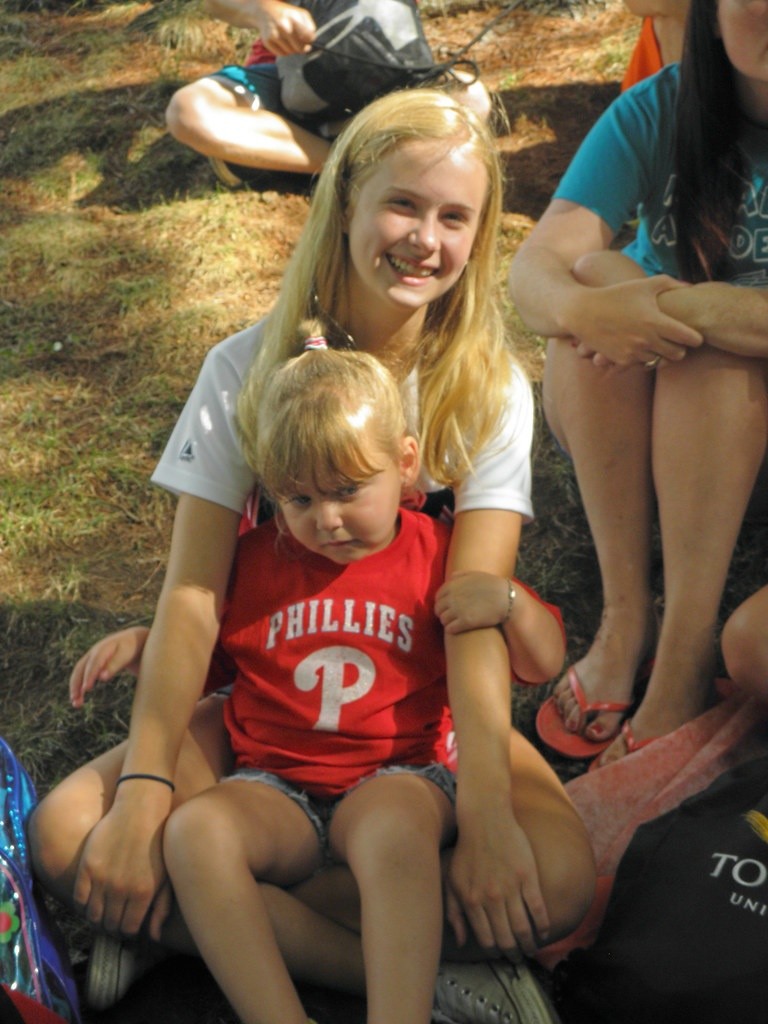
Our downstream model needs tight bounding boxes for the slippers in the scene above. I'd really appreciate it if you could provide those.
[588,717,666,778]
[535,663,635,760]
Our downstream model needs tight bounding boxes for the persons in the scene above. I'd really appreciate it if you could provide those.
[508,0,768,779]
[718,570,768,705]
[26,88,598,1024]
[65,326,570,1024]
[163,1,494,188]
[620,0,715,96]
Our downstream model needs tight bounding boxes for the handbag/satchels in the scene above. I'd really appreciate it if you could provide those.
[275,1,437,123]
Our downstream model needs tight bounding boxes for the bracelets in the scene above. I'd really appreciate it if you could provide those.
[109,768,180,791]
[501,574,515,628]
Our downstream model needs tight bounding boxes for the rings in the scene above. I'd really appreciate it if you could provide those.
[641,354,662,369]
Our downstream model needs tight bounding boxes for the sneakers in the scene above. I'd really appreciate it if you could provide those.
[82,932,141,1010]
[431,958,561,1022]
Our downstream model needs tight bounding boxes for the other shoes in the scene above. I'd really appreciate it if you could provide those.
[208,158,268,189]
